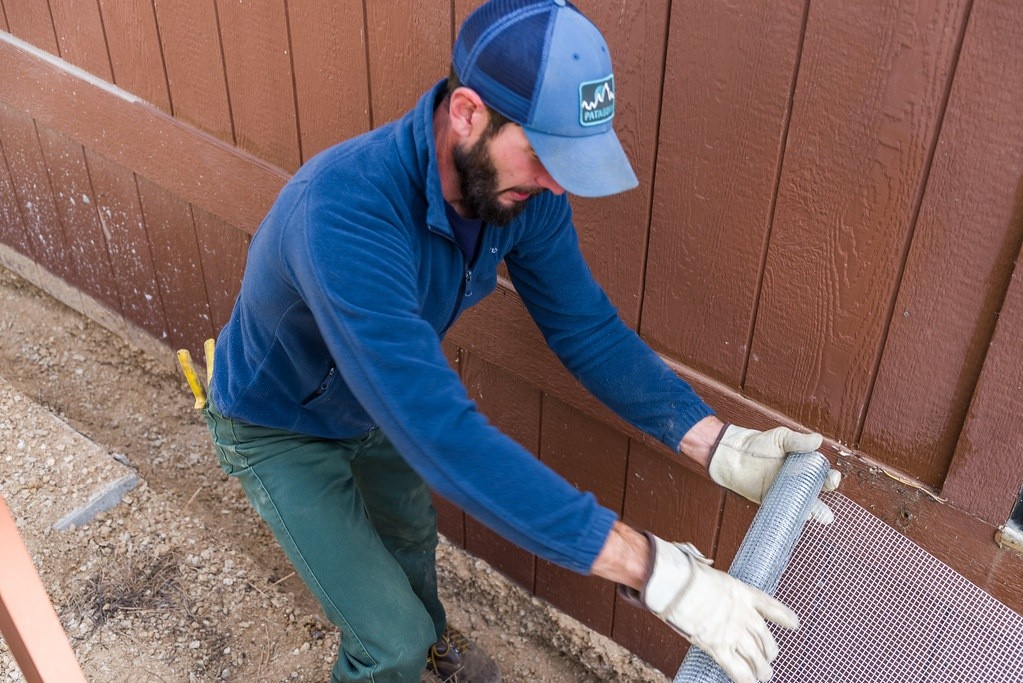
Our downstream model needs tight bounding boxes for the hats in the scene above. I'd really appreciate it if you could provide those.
[451,0,638,198]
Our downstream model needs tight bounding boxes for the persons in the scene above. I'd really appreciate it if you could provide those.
[203,0,841,683]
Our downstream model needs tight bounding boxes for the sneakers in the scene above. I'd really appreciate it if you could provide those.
[426,621,502,683]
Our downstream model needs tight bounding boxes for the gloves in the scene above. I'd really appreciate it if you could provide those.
[706,422,842,526]
[616,529,800,683]
